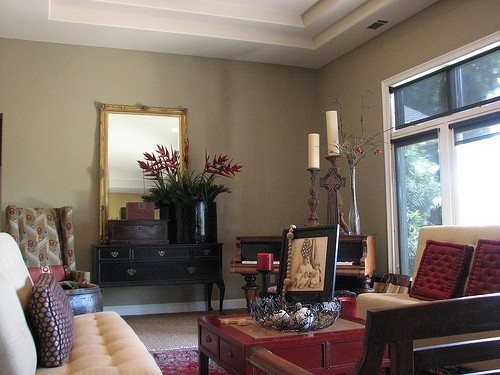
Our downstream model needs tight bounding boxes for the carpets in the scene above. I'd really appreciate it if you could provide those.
[148,347,228,375]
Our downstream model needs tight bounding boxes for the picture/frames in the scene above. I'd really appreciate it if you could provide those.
[280,224,340,301]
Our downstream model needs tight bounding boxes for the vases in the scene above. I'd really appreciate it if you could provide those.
[348,165,360,234]
[57,281,104,316]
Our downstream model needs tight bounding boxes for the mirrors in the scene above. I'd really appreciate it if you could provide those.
[97,103,188,244]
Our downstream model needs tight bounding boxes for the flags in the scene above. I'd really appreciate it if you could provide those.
[301,238,321,270]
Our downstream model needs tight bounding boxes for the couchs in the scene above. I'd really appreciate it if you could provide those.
[356,224,500,371]
[0,232,162,375]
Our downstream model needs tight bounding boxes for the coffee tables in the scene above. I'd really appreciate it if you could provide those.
[198,313,389,375]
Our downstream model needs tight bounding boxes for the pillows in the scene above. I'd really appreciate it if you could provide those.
[409,239,500,301]
[26,272,75,368]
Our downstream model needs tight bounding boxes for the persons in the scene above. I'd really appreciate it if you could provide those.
[294,258,323,289]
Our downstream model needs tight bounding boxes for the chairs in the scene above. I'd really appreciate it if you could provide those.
[6,205,90,285]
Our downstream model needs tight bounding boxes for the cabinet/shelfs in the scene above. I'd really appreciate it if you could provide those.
[90,243,226,316]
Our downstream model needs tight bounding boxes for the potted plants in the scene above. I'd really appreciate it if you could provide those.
[144,144,244,244]
[137,151,175,243]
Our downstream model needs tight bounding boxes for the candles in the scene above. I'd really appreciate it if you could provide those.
[326,111,339,156]
[308,133,320,168]
[256,253,274,270]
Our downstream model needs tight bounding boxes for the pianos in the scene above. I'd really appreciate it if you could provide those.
[230,233,377,313]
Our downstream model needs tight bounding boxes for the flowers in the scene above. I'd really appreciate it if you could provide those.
[334,94,394,233]
